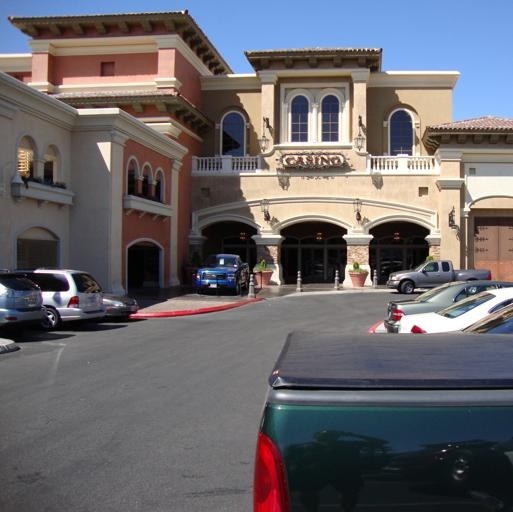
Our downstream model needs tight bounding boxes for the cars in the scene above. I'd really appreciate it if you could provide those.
[0,272,47,333]
[396,286,512,333]
[14,268,107,331]
[383,280,511,334]
[253,329,512,510]
[102,292,138,322]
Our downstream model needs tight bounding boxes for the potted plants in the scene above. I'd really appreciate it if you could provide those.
[348,262,369,288]
[252,256,273,285]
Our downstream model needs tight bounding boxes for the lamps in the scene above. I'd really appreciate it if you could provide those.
[260,199,271,221]
[353,199,362,220]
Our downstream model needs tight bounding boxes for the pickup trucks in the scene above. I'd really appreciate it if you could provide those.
[192,254,249,296]
[386,259,491,294]
